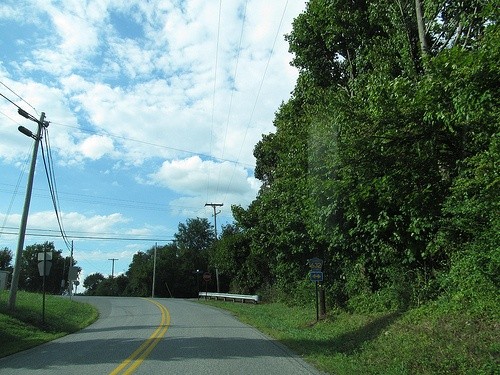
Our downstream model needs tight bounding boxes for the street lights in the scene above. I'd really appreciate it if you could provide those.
[6,109,49,310]
[150,242,159,297]
